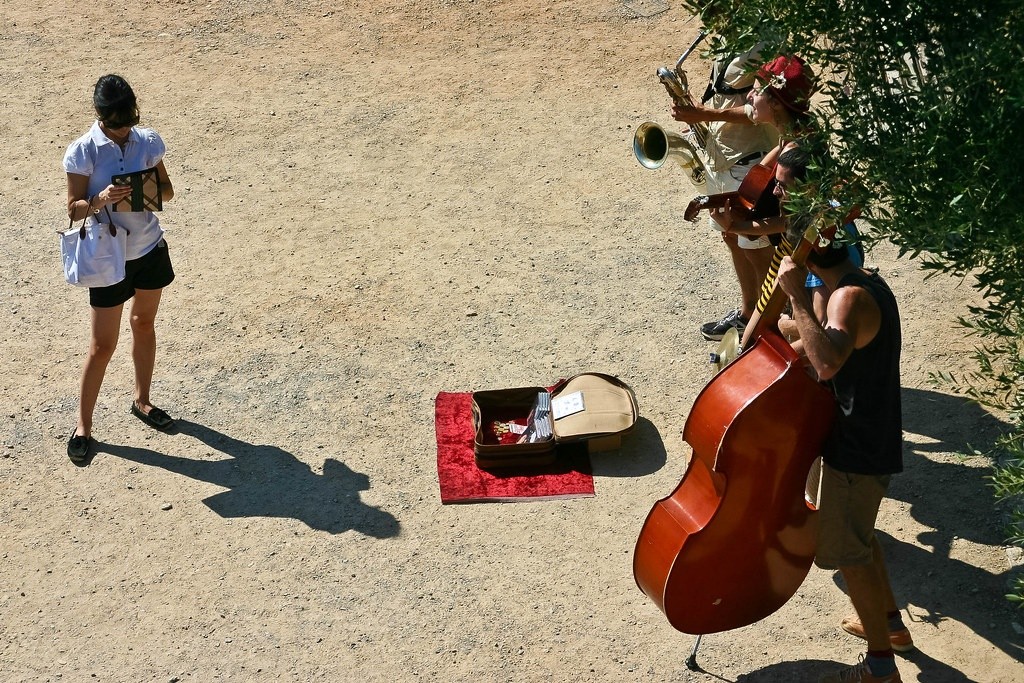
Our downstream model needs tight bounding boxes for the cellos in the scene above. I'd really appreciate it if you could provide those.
[630,175,872,668]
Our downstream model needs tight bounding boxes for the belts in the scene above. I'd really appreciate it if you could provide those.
[741,152,770,163]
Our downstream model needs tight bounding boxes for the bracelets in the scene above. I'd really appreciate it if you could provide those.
[725,221,735,234]
[88,196,100,214]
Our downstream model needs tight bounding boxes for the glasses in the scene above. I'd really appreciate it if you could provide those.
[773,179,791,193]
[101,109,142,129]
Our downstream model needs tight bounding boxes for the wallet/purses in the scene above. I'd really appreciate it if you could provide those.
[111,167,164,213]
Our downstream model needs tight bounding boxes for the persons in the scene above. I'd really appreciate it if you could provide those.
[671,19,788,358]
[709,54,865,507]
[777,202,913,683]
[63,73,176,463]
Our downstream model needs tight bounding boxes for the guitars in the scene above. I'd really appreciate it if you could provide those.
[683,162,780,247]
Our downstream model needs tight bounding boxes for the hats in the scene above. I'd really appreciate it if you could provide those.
[753,56,815,112]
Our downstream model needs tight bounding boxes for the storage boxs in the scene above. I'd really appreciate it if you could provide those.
[471,372,639,465]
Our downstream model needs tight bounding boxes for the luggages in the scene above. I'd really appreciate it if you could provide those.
[470,372,640,470]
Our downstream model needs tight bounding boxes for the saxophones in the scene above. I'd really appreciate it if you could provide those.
[632,28,710,195]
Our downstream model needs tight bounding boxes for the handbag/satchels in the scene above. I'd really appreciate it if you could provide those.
[57,193,131,282]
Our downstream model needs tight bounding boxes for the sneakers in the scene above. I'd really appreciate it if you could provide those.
[66,426,91,462]
[818,656,902,683]
[131,400,174,430]
[842,614,915,650]
[699,307,749,342]
